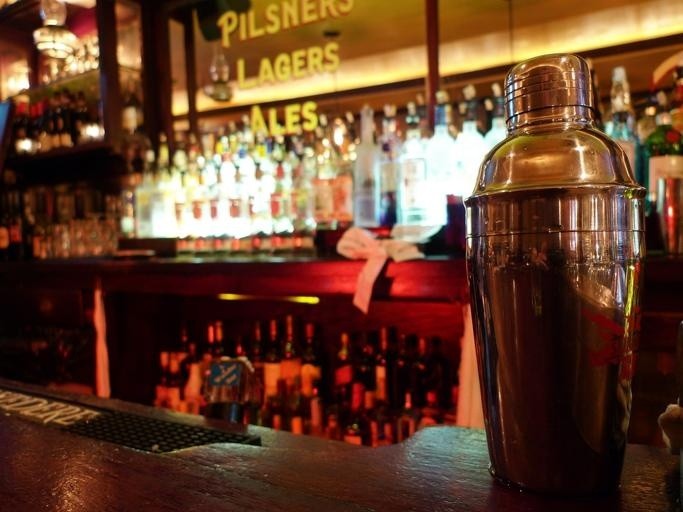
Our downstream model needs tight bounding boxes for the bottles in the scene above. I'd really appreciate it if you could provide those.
[131,306,462,451]
[459,53,647,499]
[115,58,682,240]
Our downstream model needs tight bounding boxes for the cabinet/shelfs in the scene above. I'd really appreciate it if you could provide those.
[0,0,683,447]
